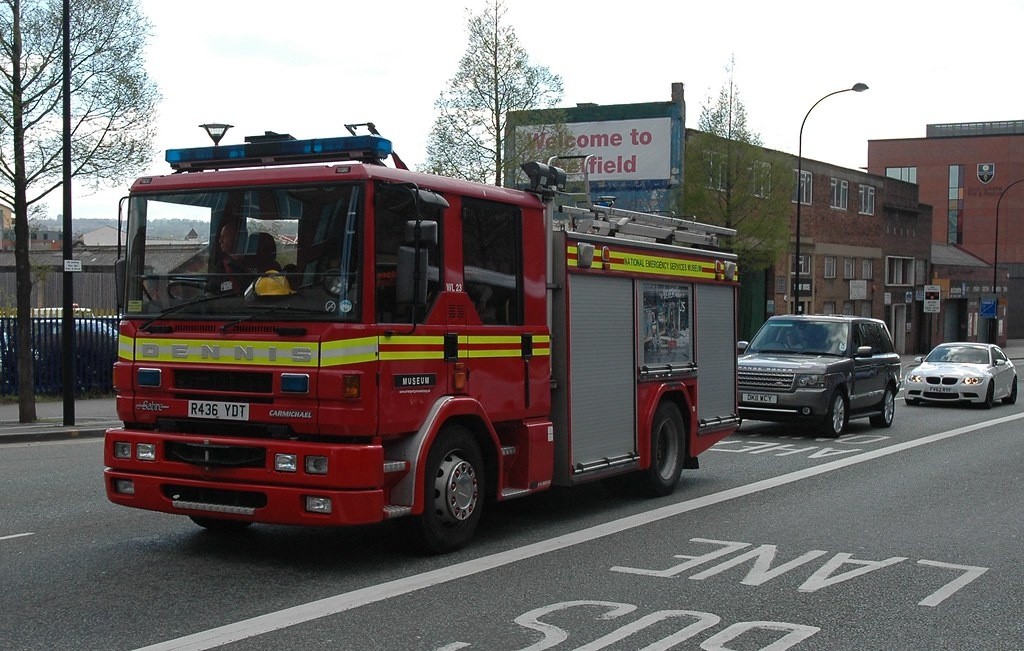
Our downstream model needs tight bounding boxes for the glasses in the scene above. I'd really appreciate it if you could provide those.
[787,334,794,336]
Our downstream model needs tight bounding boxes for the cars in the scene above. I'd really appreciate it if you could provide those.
[0,315,122,393]
[904,341,1017,409]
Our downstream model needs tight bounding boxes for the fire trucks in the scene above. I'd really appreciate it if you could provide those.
[102,132,742,550]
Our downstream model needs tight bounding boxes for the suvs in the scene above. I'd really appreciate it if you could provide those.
[738,314,902,439]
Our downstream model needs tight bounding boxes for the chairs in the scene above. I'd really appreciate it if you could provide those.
[247,232,282,272]
[464,230,486,268]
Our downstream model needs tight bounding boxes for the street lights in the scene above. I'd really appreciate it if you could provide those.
[199,123,234,172]
[795,82,868,315]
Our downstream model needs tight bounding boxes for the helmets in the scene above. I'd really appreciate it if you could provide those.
[255,271,297,296]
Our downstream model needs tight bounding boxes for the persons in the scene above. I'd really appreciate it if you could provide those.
[204,223,279,297]
[786,330,803,350]
